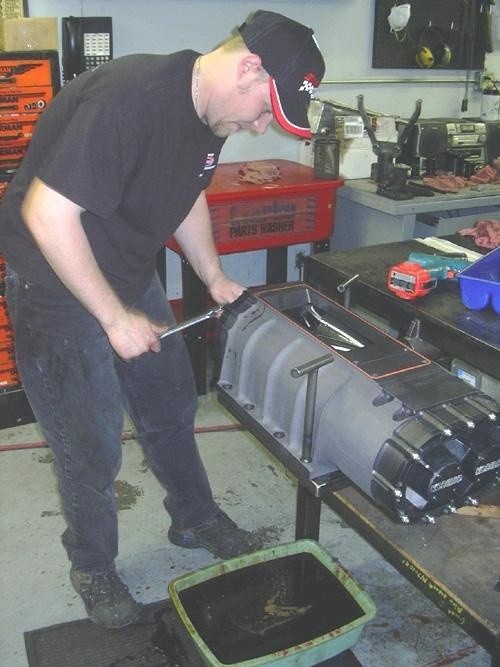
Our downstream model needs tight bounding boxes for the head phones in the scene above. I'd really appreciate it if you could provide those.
[416,25,452,68]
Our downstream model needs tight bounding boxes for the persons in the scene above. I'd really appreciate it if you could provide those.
[0,9,324,630]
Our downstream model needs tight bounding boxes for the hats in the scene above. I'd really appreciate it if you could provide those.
[238,9,325,140]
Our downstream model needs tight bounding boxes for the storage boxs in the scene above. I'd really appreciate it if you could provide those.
[167,538,374,666]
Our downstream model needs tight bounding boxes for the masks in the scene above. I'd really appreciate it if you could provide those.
[388,0,411,42]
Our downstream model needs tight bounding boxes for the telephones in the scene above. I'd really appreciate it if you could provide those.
[61,16,113,85]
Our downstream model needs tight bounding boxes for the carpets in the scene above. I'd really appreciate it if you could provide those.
[23,596,365,667]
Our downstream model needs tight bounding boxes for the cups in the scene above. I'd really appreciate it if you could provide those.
[315,140,340,180]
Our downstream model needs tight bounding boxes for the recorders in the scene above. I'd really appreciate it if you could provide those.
[396,116,500,179]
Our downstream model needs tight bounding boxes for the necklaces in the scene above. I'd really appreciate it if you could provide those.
[192,53,198,117]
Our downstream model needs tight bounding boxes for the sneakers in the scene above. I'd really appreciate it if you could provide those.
[70,561,142,631]
[168,508,262,560]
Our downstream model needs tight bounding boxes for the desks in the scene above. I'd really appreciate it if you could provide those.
[156,157,345,396]
[337,180,500,250]
[306,233,500,379]
